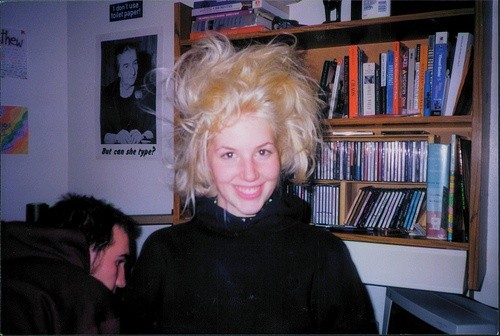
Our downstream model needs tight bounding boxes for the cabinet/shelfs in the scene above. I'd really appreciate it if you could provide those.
[173,0,484,288]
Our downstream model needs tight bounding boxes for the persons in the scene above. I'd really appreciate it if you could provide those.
[124,31,379,336]
[0,193,138,336]
[100,44,155,145]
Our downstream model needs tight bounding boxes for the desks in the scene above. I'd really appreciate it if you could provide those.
[380,286,500,336]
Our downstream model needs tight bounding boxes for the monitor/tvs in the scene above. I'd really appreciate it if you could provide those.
[382,286,500,335]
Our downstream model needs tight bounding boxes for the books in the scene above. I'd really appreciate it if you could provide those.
[287,135,470,241]
[317,32,473,117]
[191,1,290,35]
